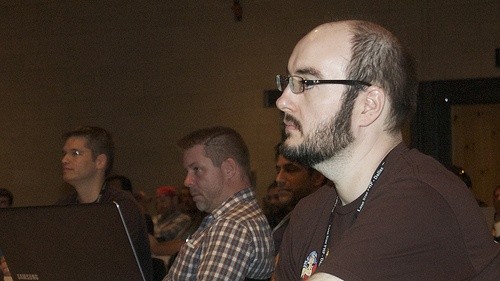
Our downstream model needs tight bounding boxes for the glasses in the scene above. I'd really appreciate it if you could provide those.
[275,73,373,96]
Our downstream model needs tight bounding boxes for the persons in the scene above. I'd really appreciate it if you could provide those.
[272,20,500,281]
[0,126,500,281]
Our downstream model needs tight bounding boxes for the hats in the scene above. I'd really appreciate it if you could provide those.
[155,187,180,198]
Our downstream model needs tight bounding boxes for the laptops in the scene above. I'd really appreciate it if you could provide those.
[0,201,146,281]
